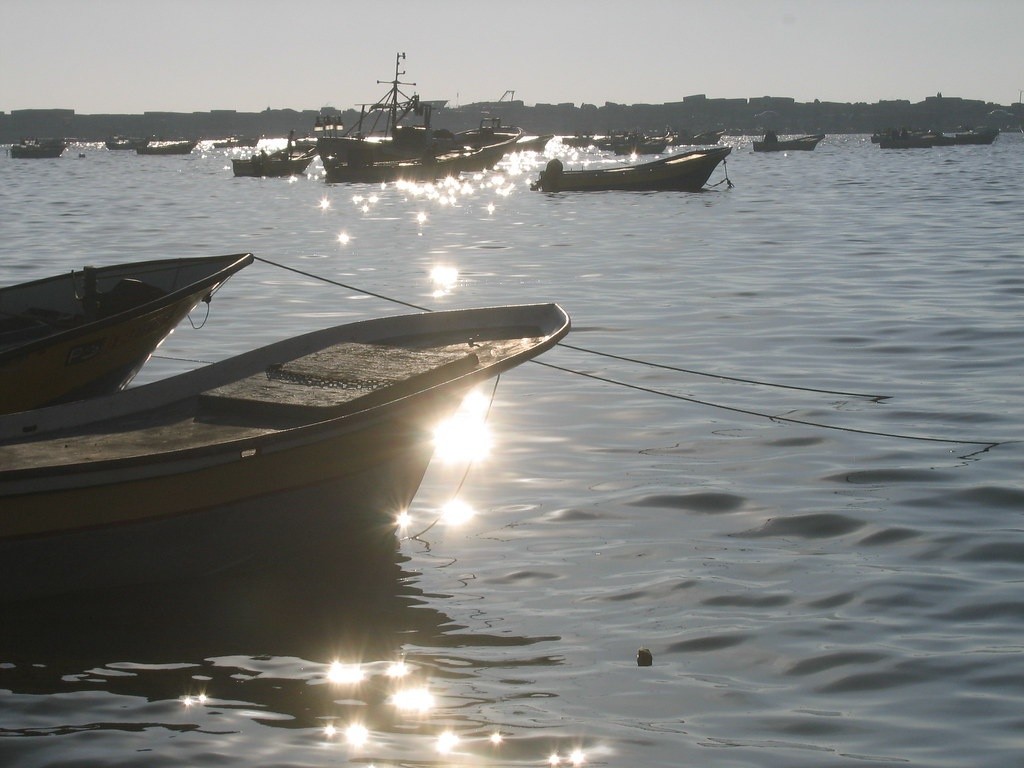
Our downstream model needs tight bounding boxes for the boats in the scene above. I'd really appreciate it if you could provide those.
[0,249,257,414]
[135,134,203,155]
[507,132,555,153]
[752,130,826,152]
[211,132,261,149]
[560,123,728,157]
[104,134,150,150]
[311,53,525,183]
[868,121,1001,149]
[527,146,733,193]
[9,136,71,158]
[230,128,319,178]
[0,297,574,545]
